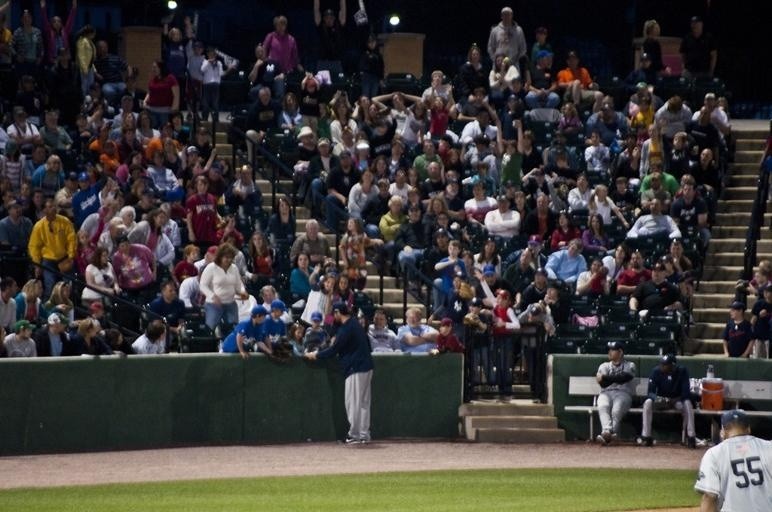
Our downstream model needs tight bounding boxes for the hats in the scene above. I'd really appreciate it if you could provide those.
[48,313,69,325]
[15,320,36,331]
[727,301,745,308]
[482,264,494,276]
[662,353,676,365]
[79,320,92,331]
[252,306,267,314]
[468,297,483,306]
[495,289,509,298]
[312,312,322,322]
[607,341,620,349]
[722,410,749,426]
[527,235,540,245]
[271,300,288,310]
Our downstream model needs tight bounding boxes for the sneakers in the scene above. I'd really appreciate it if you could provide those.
[339,436,368,445]
[636,436,654,447]
[597,432,619,446]
[683,437,707,448]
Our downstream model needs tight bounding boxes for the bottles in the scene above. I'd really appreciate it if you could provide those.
[706,364,715,377]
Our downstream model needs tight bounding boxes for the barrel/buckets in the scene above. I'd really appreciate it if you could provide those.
[700,377,726,411]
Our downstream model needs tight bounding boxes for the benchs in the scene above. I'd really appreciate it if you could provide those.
[564,376,771,445]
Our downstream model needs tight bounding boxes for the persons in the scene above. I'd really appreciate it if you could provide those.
[694,409,772,512]
[1,0,772,448]
